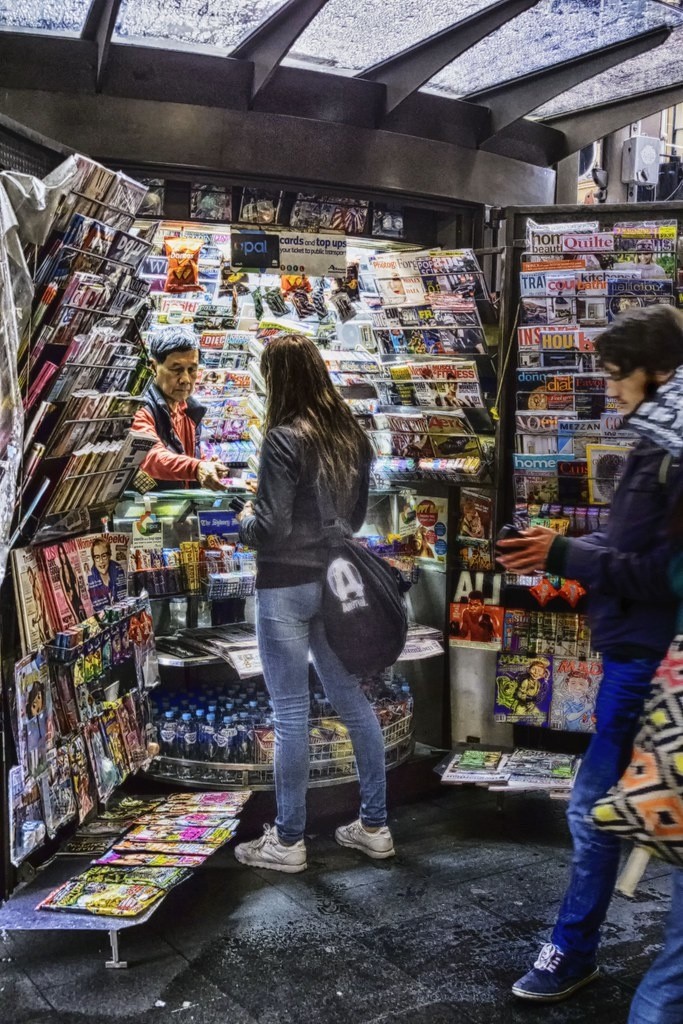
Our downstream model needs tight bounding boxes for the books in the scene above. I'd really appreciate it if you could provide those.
[1,151,255,920]
[255,215,683,801]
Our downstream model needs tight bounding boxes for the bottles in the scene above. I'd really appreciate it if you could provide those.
[151,670,413,763]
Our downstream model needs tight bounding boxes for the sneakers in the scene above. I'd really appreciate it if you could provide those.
[333,817,397,860]
[232,823,309,874]
[511,942,602,1003]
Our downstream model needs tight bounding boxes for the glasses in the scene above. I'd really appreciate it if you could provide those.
[607,370,632,381]
[91,552,108,560]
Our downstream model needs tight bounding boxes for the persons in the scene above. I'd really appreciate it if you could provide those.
[626,865,683,1024]
[130,326,232,493]
[233,334,396,874]
[495,302,682,1004]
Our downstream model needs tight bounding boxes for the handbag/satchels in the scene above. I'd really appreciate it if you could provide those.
[319,531,411,676]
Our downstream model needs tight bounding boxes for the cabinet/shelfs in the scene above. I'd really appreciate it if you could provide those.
[111,476,462,823]
